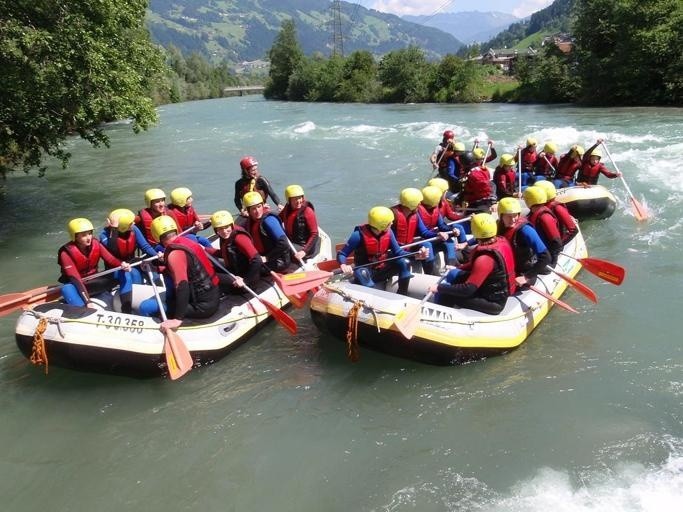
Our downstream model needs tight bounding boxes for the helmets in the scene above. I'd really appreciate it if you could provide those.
[286,185,304,200]
[500,154,515,166]
[544,144,554,153]
[368,207,394,231]
[151,216,177,242]
[444,131,453,138]
[524,186,547,208]
[498,197,521,215]
[212,211,234,230]
[471,214,497,239]
[534,181,556,200]
[528,138,536,145]
[145,189,166,208]
[171,188,192,207]
[401,188,423,210]
[422,187,442,207]
[240,157,257,169]
[572,146,584,156]
[453,144,464,151]
[473,149,484,159]
[591,150,601,157]
[69,218,95,242]
[429,179,448,192]
[243,192,263,207]
[109,209,134,233]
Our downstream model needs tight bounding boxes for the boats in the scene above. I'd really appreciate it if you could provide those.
[14,224,333,380]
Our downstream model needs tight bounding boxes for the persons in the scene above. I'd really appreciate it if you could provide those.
[197,210,264,294]
[233,155,285,226]
[234,191,290,276]
[99,208,164,287]
[335,132,621,315]
[133,188,183,253]
[167,187,214,248]
[139,216,221,334]
[264,184,319,262]
[56,217,133,313]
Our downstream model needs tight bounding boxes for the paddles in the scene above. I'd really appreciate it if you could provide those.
[198,206,281,225]
[262,261,307,308]
[145,263,193,380]
[0,266,124,317]
[286,234,328,295]
[558,252,625,286]
[599,140,648,221]
[393,268,450,340]
[546,265,596,303]
[281,248,428,296]
[317,234,442,272]
[204,250,296,335]
[523,283,579,315]
[20,253,164,312]
